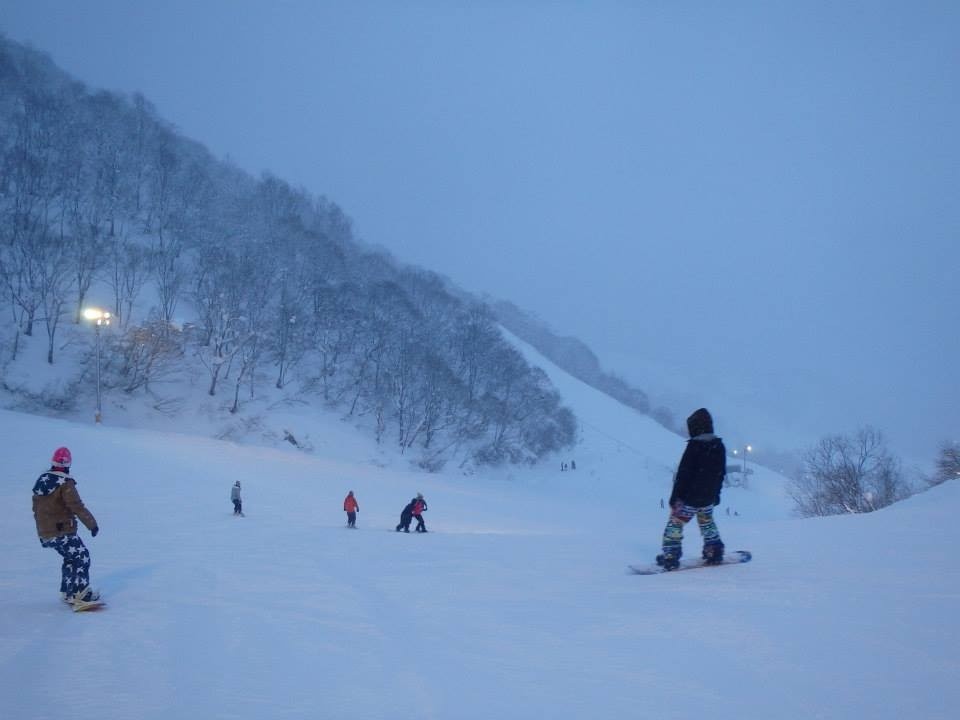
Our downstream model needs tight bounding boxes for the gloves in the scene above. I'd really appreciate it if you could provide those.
[713,496,720,507]
[92,527,99,537]
[669,496,677,506]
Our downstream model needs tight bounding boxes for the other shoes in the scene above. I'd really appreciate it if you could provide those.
[89,592,101,601]
[655,555,679,570]
[702,550,723,564]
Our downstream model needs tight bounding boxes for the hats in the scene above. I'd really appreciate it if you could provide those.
[49,447,72,469]
[235,480,241,487]
[416,491,424,498]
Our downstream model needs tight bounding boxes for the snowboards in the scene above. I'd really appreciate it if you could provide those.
[627,550,751,575]
[63,597,107,612]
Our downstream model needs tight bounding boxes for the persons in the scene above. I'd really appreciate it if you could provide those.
[396,494,428,532]
[33,447,100,603]
[344,491,359,528]
[656,408,726,566]
[231,480,242,513]
